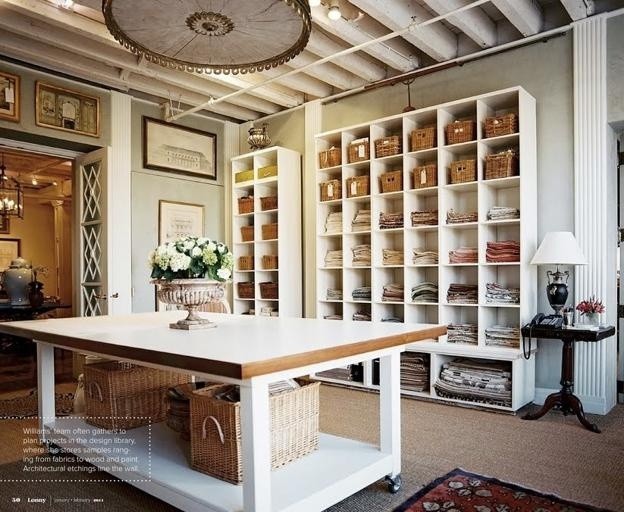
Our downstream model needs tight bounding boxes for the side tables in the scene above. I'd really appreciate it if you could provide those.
[521,326,615,434]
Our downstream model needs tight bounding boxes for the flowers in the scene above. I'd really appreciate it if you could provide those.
[31,262,48,280]
[149,234,234,282]
[576,295,606,315]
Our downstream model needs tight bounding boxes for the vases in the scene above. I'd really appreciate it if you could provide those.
[28,280,44,308]
[150,277,227,332]
[582,314,603,327]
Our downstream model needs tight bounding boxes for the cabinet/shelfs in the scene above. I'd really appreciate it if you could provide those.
[230,147,302,318]
[313,86,539,420]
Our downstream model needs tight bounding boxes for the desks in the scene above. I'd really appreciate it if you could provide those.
[0,312,446,509]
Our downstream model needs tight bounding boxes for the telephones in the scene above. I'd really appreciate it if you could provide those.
[524,313,564,360]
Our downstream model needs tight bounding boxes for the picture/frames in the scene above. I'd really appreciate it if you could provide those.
[0,238,22,258]
[141,113,218,180]
[157,199,206,243]
[1,216,10,234]
[34,80,101,138]
[0,70,22,123]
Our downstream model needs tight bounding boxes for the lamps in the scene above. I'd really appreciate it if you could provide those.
[328,0,341,19]
[100,0,311,75]
[531,230,585,315]
[0,170,25,219]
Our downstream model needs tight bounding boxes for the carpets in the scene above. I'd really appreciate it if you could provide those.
[390,466,606,512]
